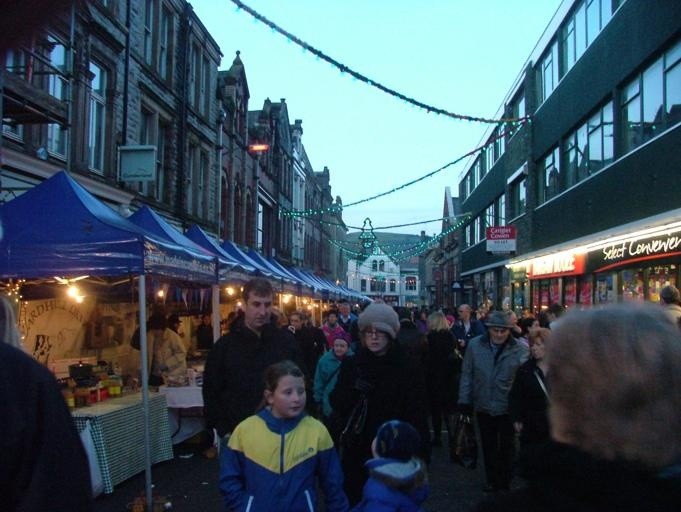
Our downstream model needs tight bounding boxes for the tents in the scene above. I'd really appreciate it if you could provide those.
[1,170,221,512]
[116,204,376,324]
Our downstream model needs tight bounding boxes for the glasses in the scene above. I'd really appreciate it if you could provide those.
[364,330,387,337]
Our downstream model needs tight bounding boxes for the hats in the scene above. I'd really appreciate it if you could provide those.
[660,286,680,299]
[484,310,515,329]
[333,330,352,347]
[358,299,400,339]
[375,421,422,462]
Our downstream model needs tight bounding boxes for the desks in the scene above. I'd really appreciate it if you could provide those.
[66,391,175,497]
[160,385,207,442]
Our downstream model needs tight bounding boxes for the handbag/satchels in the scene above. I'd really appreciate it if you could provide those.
[452,414,478,469]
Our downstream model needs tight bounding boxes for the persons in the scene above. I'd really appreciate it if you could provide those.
[147,317,187,386]
[131,311,163,351]
[656,287,679,322]
[338,302,359,333]
[474,303,679,511]
[351,421,428,511]
[330,299,428,507]
[443,302,480,348]
[168,315,185,339]
[509,327,553,506]
[202,279,301,451]
[1,300,93,507]
[422,310,459,450]
[196,314,213,345]
[217,363,349,512]
[279,311,314,345]
[486,294,576,338]
[321,310,351,350]
[312,332,357,452]
[391,302,424,354]
[459,310,530,501]
